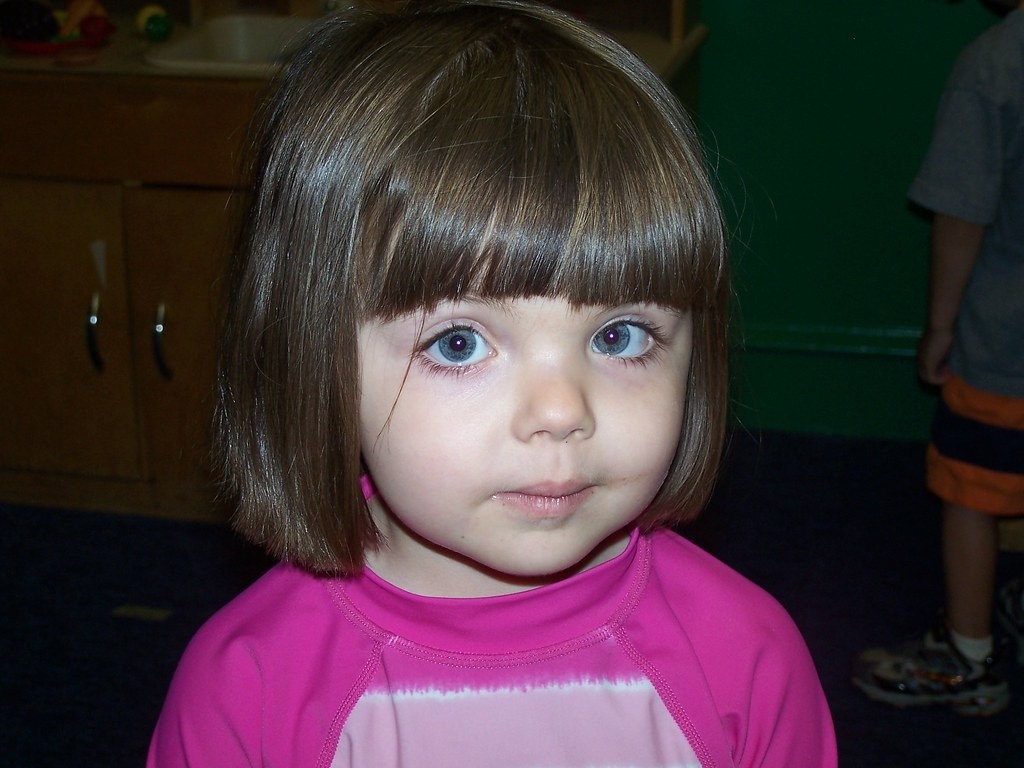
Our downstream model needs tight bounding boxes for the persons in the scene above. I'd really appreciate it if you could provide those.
[140,0,839,768]
[852,0,1024,719]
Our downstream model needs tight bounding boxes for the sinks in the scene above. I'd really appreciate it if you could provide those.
[126,12,331,79]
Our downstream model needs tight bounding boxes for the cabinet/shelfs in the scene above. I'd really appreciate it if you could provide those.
[1,68,270,522]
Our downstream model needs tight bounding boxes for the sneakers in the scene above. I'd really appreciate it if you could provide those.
[995,578,1024,652]
[843,621,1012,721]
